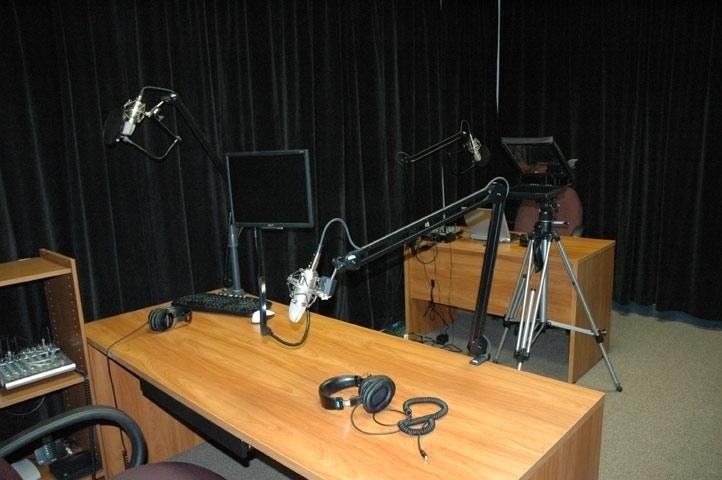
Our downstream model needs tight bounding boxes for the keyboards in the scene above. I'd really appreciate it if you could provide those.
[172,291,272,316]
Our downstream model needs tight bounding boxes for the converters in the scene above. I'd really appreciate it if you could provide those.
[436,334,448,344]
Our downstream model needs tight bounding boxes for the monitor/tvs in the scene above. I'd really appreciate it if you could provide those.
[224,149,317,231]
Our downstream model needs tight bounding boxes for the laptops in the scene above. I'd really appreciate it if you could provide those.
[461,206,520,243]
[500,136,575,201]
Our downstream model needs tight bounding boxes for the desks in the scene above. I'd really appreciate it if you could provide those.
[404,236,619,382]
[84,285,606,480]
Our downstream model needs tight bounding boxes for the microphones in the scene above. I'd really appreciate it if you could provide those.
[468,133,481,162]
[290,245,323,323]
[118,94,144,136]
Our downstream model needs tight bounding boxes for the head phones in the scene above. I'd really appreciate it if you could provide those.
[148,306,192,331]
[320,374,395,414]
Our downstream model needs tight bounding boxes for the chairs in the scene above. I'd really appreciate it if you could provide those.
[515,185,584,239]
[1,404,230,479]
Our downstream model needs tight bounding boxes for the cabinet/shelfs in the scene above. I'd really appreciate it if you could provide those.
[1,241,111,479]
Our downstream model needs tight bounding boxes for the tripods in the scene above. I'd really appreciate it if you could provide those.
[494,199,622,392]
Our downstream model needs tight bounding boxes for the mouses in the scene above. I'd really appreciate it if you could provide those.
[250,309,275,323]
